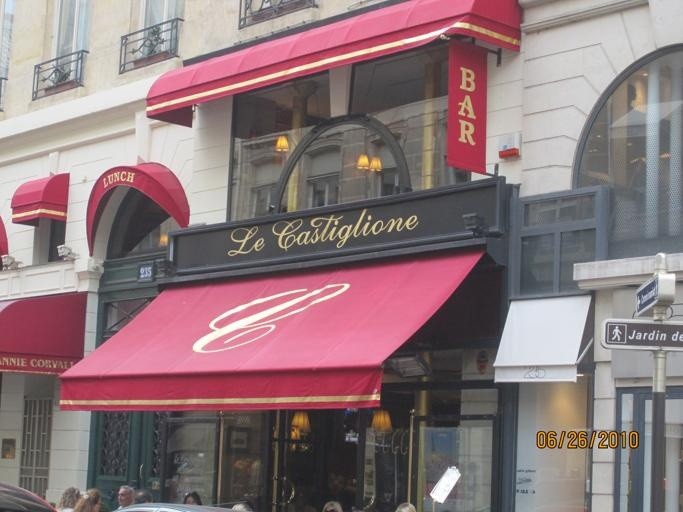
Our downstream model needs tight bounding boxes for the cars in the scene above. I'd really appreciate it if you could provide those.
[113,503,249,512]
[0,479,57,512]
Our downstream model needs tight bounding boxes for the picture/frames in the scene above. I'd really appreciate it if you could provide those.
[226,424,254,453]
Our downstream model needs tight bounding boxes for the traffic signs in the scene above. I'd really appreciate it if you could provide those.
[634,269,674,314]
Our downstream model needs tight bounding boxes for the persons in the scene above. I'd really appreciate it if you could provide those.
[57,486,80,512]
[74,488,101,512]
[183,491,201,505]
[116,484,134,509]
[395,502,416,512]
[132,488,152,503]
[232,502,253,512]
[321,500,343,511]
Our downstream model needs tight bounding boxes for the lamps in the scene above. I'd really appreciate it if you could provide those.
[356,129,384,173]
[1,255,19,272]
[276,135,289,163]
[56,245,78,260]
